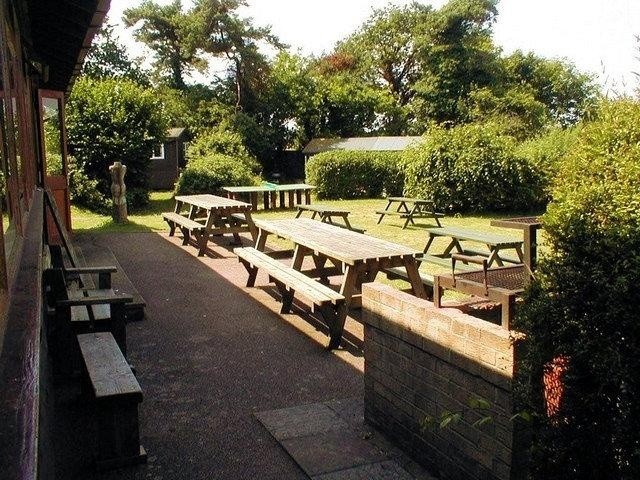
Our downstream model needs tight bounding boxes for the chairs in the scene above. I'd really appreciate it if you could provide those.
[48,243,134,354]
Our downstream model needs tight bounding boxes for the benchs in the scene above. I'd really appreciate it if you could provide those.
[77,331,148,464]
